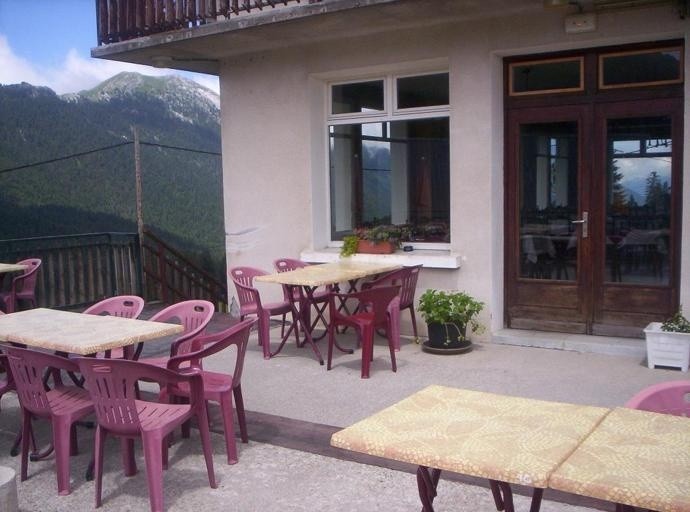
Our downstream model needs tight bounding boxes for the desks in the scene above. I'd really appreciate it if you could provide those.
[330,383,611,512]
[547,406,690,512]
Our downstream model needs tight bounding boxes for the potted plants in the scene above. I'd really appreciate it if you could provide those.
[416,289,489,355]
[642,303,690,372]
[339,224,403,257]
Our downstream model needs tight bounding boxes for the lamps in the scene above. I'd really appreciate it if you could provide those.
[543,0,598,34]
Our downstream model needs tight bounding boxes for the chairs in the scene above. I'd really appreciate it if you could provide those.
[624,381,690,419]
